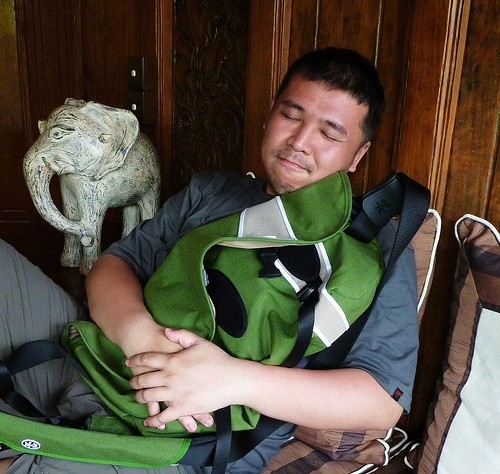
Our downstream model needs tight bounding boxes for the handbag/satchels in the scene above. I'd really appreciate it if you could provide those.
[0,170,432,474]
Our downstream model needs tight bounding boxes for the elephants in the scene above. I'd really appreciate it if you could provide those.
[23,97,161,275]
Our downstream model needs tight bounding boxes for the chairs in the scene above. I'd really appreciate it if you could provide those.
[403,211,500,474]
[256,206,444,474]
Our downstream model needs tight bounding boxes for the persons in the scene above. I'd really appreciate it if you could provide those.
[1,46,419,474]
